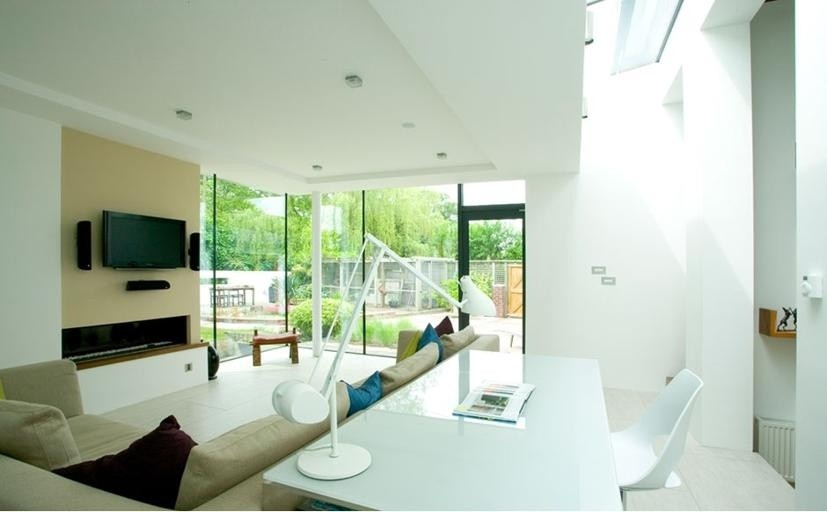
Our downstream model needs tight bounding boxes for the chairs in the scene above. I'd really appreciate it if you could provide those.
[608,367,704,511]
[215,286,243,307]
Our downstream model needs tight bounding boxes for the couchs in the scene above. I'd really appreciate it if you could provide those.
[0,328,500,510]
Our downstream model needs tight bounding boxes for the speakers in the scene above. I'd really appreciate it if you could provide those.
[127,281,171,291]
[188,232,201,271]
[77,220,92,270]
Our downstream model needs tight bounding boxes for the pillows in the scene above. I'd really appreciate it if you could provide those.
[397,316,456,366]
[338,369,383,418]
[51,413,200,510]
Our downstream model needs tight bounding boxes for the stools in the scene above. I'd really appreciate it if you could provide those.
[251,326,300,366]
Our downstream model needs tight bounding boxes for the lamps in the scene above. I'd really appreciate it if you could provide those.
[272,232,499,482]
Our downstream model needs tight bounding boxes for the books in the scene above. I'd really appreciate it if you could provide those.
[451,381,537,423]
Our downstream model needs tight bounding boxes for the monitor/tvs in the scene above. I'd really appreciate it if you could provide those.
[102,210,187,271]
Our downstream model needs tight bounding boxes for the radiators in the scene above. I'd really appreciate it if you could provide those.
[756,416,798,485]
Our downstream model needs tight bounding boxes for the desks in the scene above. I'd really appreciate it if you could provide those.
[259,349,625,510]
[210,288,254,308]
